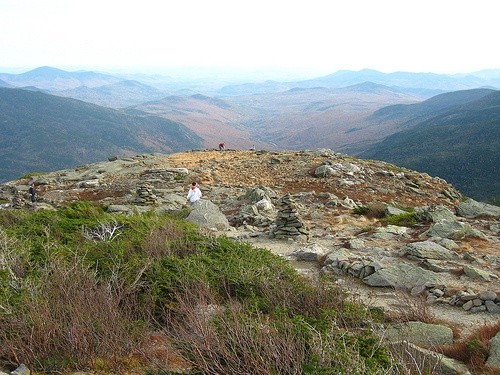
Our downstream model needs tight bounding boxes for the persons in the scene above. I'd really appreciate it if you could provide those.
[249,145,256,151]
[218,143,225,151]
[187,182,203,207]
[29,182,36,202]
